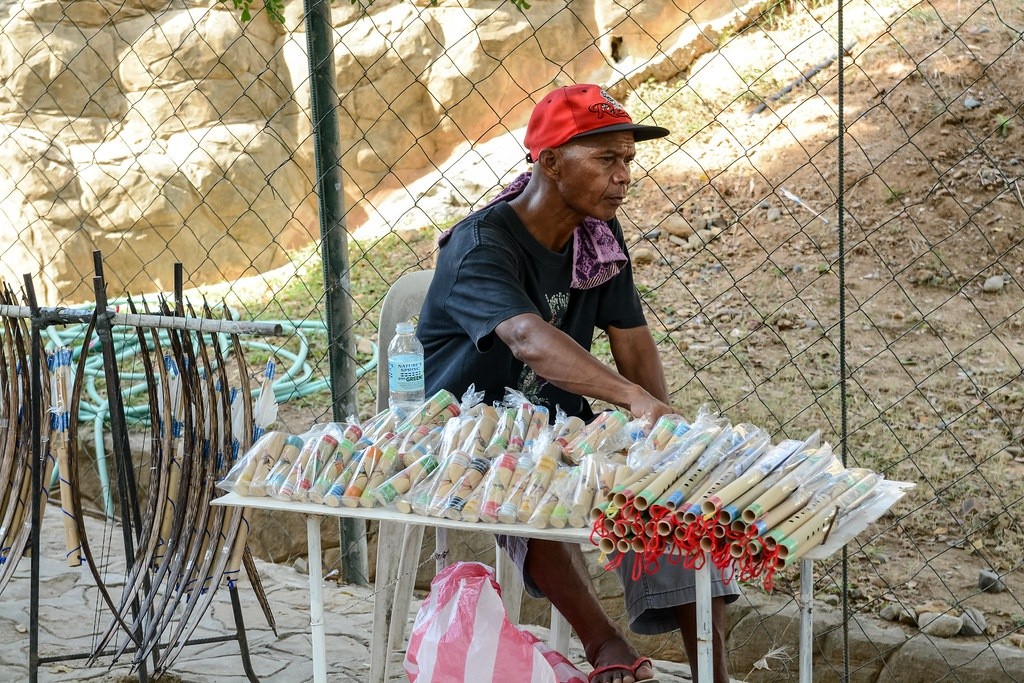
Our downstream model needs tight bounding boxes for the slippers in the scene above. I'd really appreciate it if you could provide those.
[589,657,659,683]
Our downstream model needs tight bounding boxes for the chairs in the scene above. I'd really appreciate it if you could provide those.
[368,269,571,683]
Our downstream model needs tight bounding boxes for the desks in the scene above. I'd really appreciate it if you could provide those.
[208,478,914,683]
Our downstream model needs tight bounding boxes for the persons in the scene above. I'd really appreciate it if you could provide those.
[416,83,741,683]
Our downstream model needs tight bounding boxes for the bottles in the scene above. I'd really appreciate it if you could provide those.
[387,322,426,420]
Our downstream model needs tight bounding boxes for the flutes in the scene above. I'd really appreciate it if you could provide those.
[586,420,883,571]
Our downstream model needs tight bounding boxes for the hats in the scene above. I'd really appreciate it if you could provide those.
[524,84,670,164]
